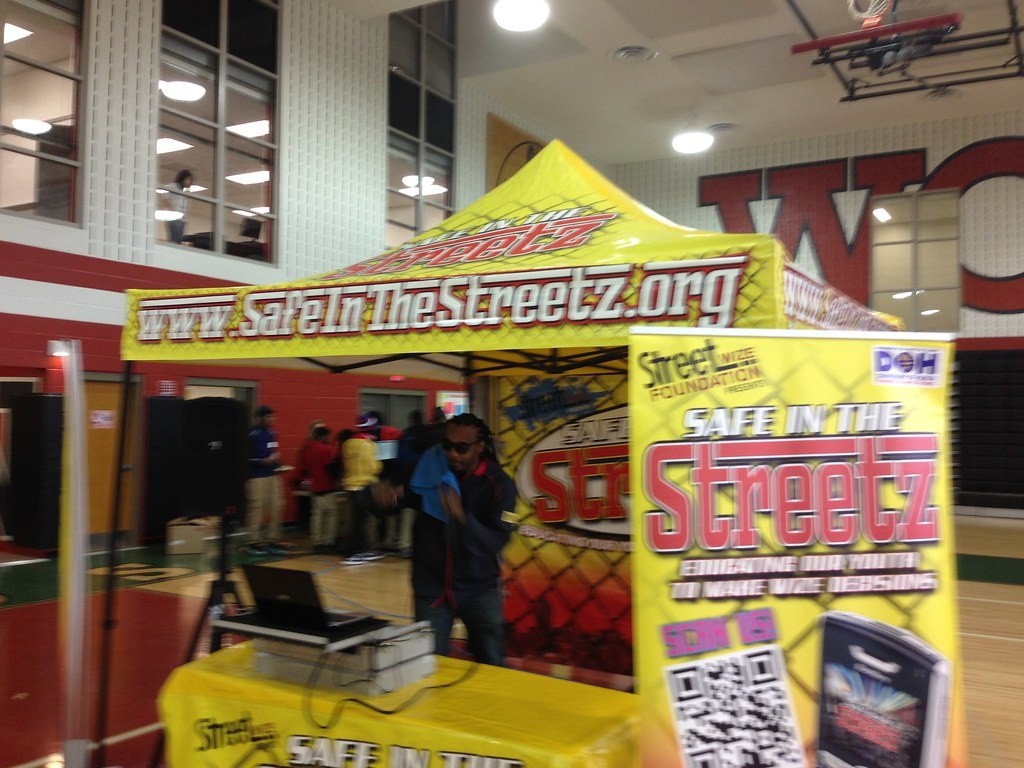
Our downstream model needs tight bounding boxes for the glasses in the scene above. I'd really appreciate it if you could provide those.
[441,437,480,455]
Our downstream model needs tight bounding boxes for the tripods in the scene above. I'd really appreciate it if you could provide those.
[147,516,249,767]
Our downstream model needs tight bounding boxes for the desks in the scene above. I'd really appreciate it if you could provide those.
[171,637,641,768]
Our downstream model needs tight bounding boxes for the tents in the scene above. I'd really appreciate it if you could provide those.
[78,137,908,768]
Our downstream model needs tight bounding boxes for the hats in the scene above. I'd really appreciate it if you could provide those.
[310,419,331,438]
[380,425,403,441]
[355,415,379,428]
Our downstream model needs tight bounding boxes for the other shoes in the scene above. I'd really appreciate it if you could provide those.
[310,541,410,565]
[268,539,300,554]
[237,542,269,557]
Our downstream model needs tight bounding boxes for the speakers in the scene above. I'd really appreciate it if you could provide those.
[171,395,250,513]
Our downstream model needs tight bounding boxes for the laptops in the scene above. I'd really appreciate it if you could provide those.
[242,564,373,631]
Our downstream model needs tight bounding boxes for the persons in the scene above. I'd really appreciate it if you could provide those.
[405,413,521,668]
[298,406,404,568]
[162,168,194,247]
[395,408,436,557]
[430,406,451,441]
[234,404,294,548]
